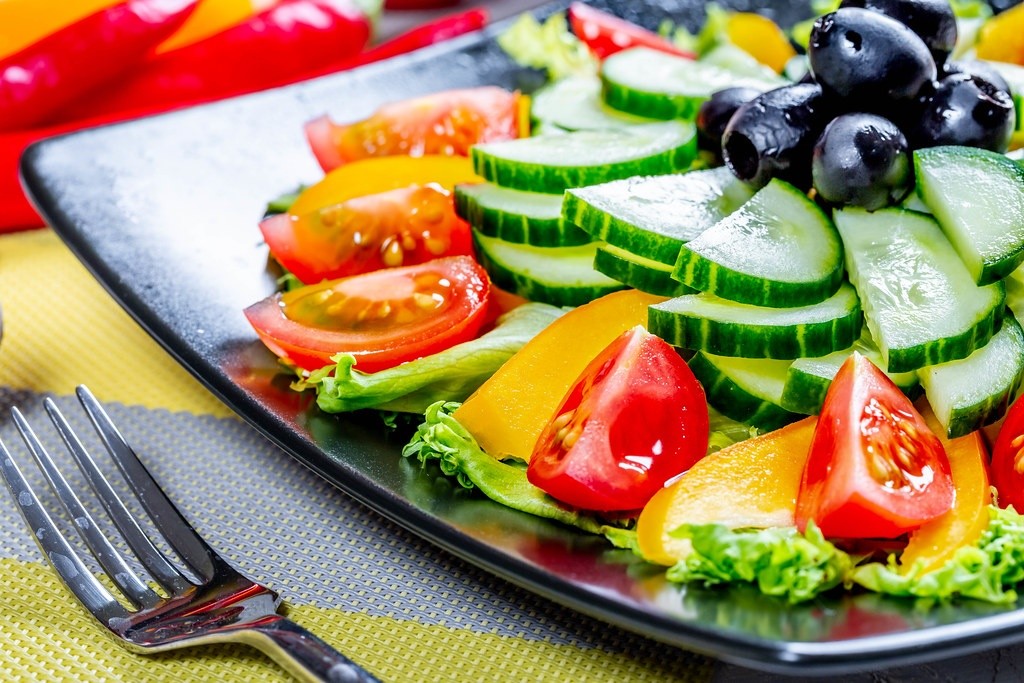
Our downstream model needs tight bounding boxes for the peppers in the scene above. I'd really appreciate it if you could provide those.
[0,0,487,235]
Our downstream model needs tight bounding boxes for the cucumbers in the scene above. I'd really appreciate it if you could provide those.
[454,35,1024,439]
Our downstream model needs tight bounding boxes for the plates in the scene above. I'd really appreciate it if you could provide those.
[17,1,1024,677]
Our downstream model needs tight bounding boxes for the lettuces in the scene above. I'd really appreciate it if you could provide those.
[665,486,1024,605]
[279,303,582,429]
[403,400,767,549]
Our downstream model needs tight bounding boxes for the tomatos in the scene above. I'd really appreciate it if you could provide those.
[794,350,955,538]
[258,182,476,285]
[990,393,1024,517]
[244,254,493,375]
[304,85,522,175]
[526,323,709,512]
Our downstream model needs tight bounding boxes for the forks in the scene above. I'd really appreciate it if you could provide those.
[1,384,388,682]
